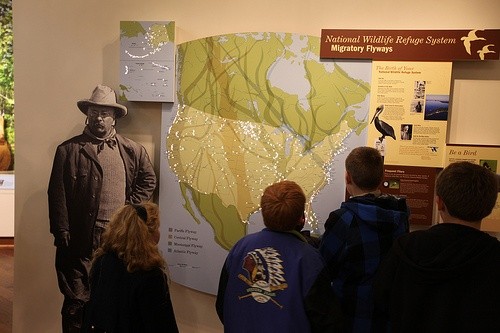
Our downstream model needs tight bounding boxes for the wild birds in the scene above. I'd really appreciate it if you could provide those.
[370,104,396,142]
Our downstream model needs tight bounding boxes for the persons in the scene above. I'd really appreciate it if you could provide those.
[312,146,413,333]
[410,160,500,333]
[214,181,338,333]
[46,85,159,333]
[88,202,181,333]
